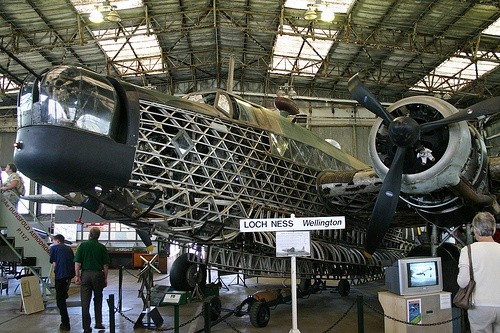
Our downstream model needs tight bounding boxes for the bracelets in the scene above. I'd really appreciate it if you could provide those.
[76,275,80,277]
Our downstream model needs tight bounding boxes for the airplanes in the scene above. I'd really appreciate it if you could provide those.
[7,62,500,327]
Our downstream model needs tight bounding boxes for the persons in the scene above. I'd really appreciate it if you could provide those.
[73,226,110,333]
[49,234,75,331]
[2,164,21,211]
[457,212,500,333]
[0,166,2,187]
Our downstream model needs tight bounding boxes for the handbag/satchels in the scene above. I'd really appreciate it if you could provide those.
[453,280,476,310]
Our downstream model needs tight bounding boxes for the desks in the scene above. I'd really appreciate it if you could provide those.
[107,251,148,270]
[141,255,158,267]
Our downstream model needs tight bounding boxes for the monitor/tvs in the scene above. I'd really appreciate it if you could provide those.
[384,256,444,296]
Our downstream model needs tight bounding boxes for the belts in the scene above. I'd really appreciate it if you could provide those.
[83,269,101,272]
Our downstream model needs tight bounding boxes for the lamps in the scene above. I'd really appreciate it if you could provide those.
[88,0,121,24]
[303,0,335,22]
[434,90,452,100]
[277,85,298,96]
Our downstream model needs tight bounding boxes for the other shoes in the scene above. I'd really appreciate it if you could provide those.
[84,328,92,333]
[95,323,106,329]
[60,323,70,331]
